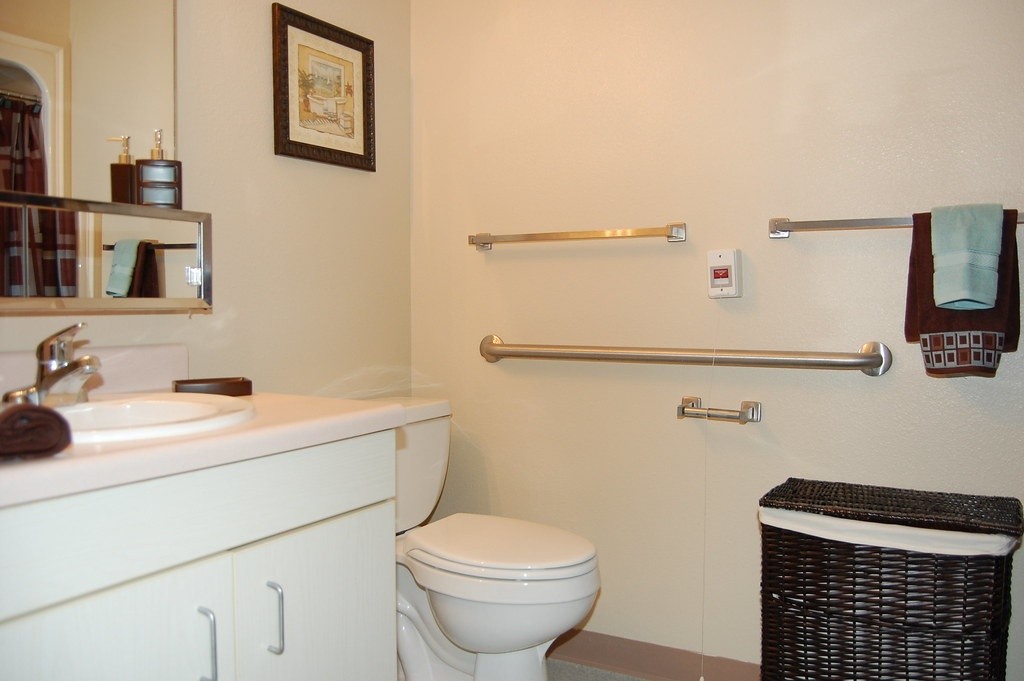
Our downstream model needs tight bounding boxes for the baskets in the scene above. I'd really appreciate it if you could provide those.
[758,478,1024,681]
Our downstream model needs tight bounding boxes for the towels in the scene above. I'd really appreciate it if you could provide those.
[127,240,161,298]
[930,202,1004,312]
[105,238,140,298]
[0,403,72,460]
[904,209,1020,378]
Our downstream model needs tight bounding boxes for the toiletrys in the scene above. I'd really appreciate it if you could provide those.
[107,134,136,202]
[136,127,181,211]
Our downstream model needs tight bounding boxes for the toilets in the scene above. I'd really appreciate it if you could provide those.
[368,395,602,679]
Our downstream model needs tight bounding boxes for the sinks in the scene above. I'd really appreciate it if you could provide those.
[54,399,218,430]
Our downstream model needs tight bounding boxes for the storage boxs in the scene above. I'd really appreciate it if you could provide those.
[756,477,1024,681]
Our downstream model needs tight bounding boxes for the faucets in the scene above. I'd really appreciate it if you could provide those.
[1,321,99,407]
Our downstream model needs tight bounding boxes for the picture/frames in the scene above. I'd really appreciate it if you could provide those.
[272,1,376,171]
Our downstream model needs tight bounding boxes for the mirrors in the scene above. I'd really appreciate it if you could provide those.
[1,189,215,314]
[1,0,178,206]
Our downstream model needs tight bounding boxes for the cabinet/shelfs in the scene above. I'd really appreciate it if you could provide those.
[0,495,397,680]
